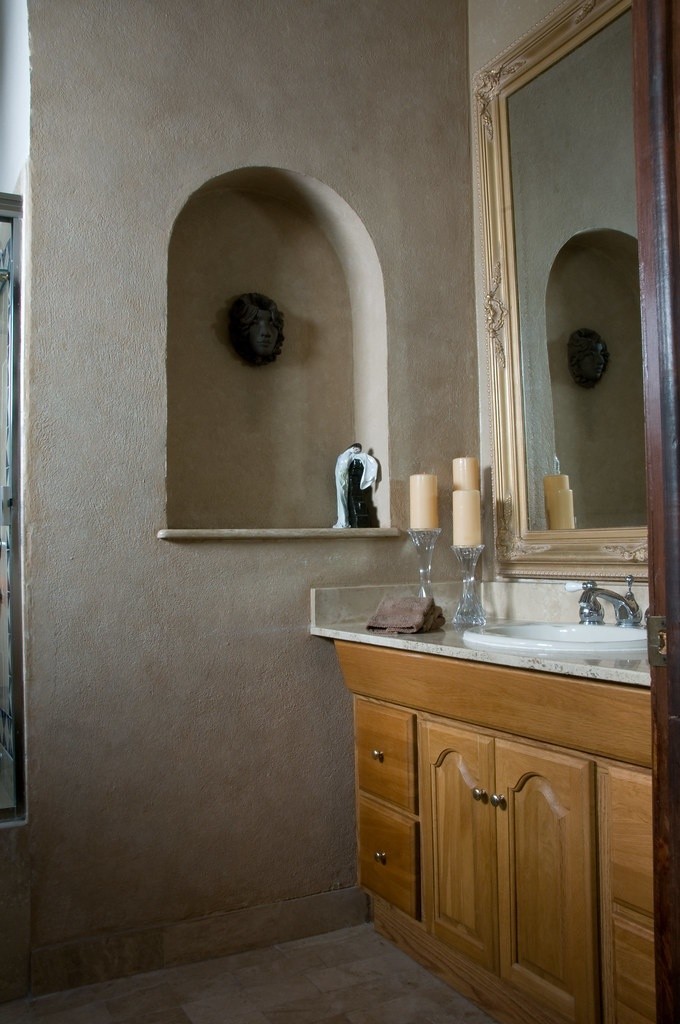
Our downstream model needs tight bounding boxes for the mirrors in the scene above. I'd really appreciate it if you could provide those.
[472,0,646,582]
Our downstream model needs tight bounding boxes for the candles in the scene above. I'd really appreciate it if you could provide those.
[453,454,480,491]
[409,471,439,531]
[452,490,482,548]
[543,453,576,529]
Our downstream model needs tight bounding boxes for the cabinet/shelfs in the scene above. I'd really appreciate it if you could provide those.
[308,618,658,1024]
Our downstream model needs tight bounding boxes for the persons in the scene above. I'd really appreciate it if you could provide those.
[568,326,610,389]
[228,292,286,367]
[333,443,379,529]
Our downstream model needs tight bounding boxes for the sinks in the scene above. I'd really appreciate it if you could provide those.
[463,622,648,652]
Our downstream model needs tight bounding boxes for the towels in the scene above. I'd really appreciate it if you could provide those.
[366,597,446,634]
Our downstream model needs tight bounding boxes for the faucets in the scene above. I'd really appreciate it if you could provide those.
[579,575,643,628]
